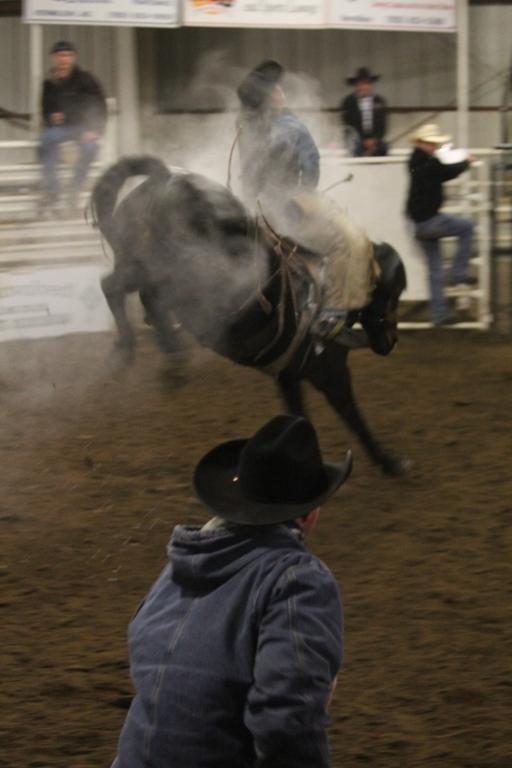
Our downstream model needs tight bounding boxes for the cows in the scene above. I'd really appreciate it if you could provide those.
[85,156,410,479]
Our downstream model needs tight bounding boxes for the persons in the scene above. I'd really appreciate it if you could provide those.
[235,59,374,349]
[110,411,356,767]
[34,37,109,216]
[340,65,392,160]
[404,121,479,330]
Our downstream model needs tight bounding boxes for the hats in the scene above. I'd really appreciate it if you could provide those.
[408,124,453,144]
[345,67,381,85]
[236,61,282,107]
[49,40,75,53]
[192,413,353,526]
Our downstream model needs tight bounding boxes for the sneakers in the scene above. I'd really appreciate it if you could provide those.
[436,314,464,326]
[448,272,476,287]
[320,317,361,346]
